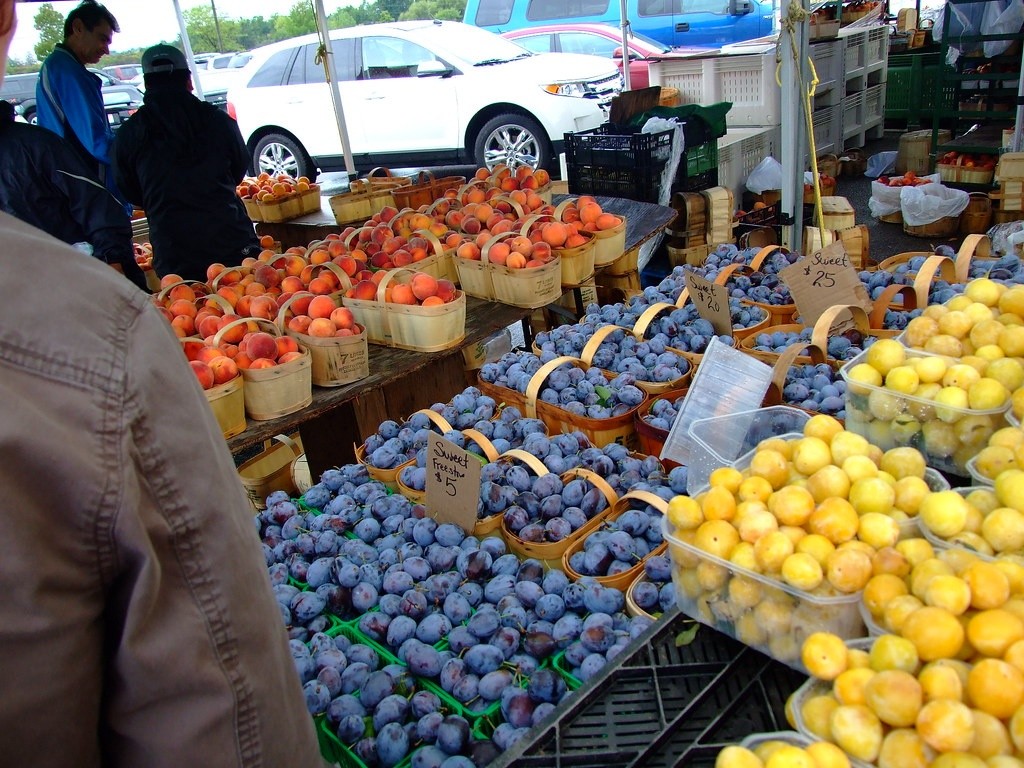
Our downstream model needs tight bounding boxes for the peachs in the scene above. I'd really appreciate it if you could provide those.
[877,171,933,187]
[803,173,835,191]
[809,0,880,25]
[939,152,999,170]
[129,164,622,391]
[736,202,766,223]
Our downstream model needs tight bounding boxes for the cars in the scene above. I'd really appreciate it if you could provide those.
[496,23,716,91]
[1,53,238,133]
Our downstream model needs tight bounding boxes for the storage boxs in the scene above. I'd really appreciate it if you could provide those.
[658,291,1024,768]
[562,85,718,207]
[733,199,814,247]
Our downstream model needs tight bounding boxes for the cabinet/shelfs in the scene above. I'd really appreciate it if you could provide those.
[929,0,1024,174]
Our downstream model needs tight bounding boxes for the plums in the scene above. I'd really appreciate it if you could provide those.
[253,243,1024,768]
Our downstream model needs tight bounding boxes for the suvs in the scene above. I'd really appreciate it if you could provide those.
[226,19,623,183]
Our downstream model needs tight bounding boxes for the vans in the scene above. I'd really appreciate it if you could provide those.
[463,0,778,47]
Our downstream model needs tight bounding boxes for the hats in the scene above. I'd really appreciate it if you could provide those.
[141,42,188,74]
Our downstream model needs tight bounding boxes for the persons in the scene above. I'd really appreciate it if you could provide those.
[0,209,328,768]
[35,0,133,220]
[111,44,262,287]
[0,0,148,293]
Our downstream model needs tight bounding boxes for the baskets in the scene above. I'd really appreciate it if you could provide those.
[255,466,652,768]
